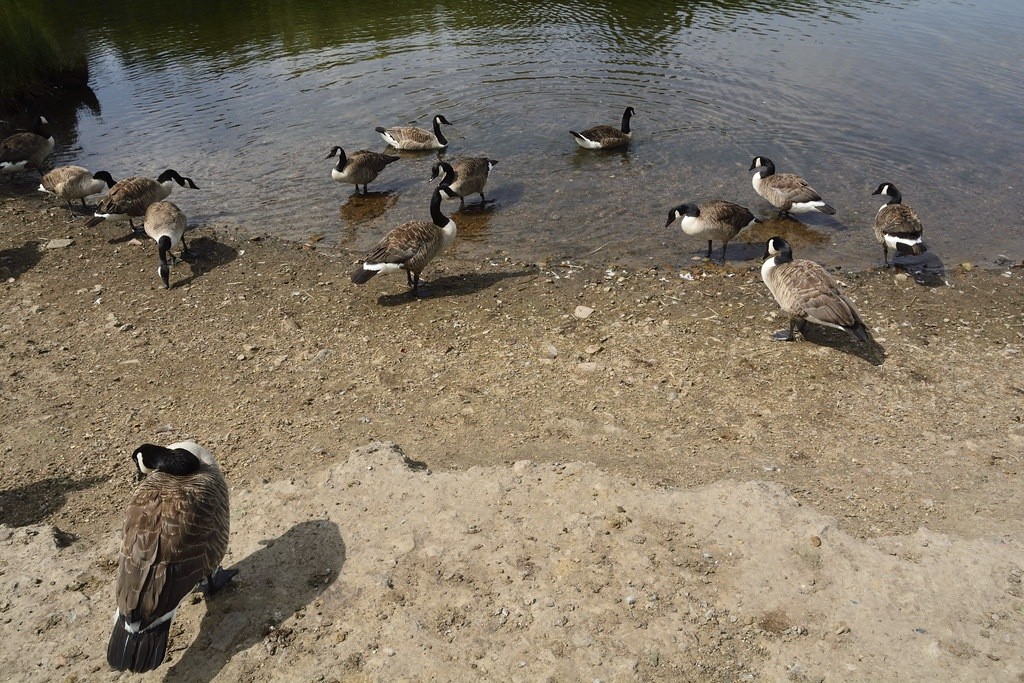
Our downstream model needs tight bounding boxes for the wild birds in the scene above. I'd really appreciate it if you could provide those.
[872,182,927,271]
[429,157,499,202]
[748,156,837,219]
[143,201,199,290]
[349,185,462,297]
[37,165,117,220]
[106,442,240,673]
[86,169,200,233]
[568,107,636,150]
[761,237,869,343]
[375,115,453,151]
[664,199,764,261]
[0,113,60,187]
[325,145,400,194]
